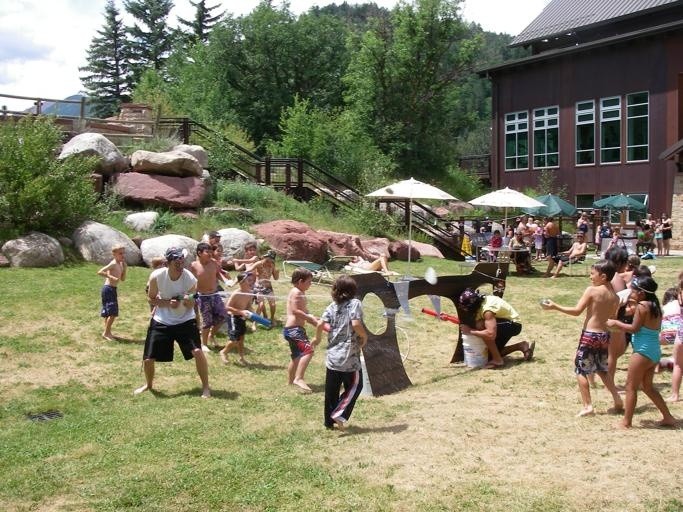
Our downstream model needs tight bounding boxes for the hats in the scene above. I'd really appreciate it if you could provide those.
[263,250,276,260]
[165,247,184,258]
[209,231,222,239]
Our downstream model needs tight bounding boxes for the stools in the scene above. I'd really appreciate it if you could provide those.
[460,263,476,275]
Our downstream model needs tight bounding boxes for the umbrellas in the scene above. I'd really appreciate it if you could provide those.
[591,192,645,211]
[363,176,458,263]
[465,185,548,240]
[521,193,578,218]
[627,196,647,211]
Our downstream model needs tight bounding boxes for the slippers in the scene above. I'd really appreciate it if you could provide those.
[523,341,535,361]
[478,363,504,370]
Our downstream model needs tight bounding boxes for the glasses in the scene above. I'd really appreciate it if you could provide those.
[631,278,638,289]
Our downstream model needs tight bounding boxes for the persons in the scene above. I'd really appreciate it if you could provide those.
[432,212,674,279]
[283,268,329,393]
[133,247,212,399]
[349,256,392,273]
[145,231,280,367]
[539,260,623,418]
[605,246,683,401]
[95,244,128,341]
[310,274,369,431]
[437,289,529,369]
[605,276,676,428]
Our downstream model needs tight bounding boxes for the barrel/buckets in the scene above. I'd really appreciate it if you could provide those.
[461,333,488,369]
[359,354,373,395]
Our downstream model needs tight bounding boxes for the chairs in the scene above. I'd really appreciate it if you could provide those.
[283,254,354,290]
[638,229,656,257]
[561,247,589,278]
[344,263,402,282]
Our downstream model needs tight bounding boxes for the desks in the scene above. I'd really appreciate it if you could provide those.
[556,236,573,253]
[481,244,531,276]
